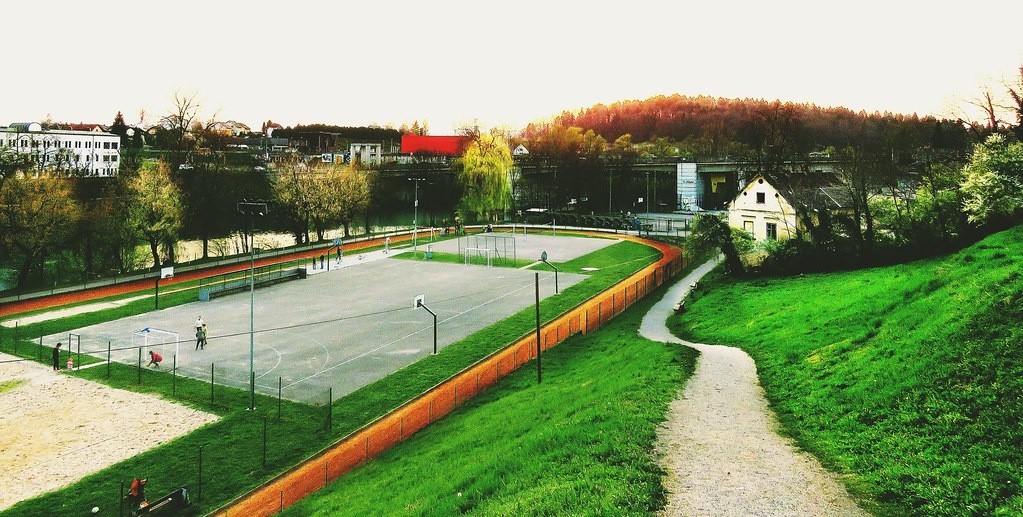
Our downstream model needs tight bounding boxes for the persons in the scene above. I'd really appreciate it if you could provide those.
[147,350,163,368]
[66,358,74,372]
[312,256,317,270]
[201,324,208,345]
[335,246,342,260]
[52,342,62,371]
[195,329,206,350]
[486,223,493,233]
[445,224,450,234]
[589,207,640,222]
[319,253,324,269]
[194,315,205,331]
